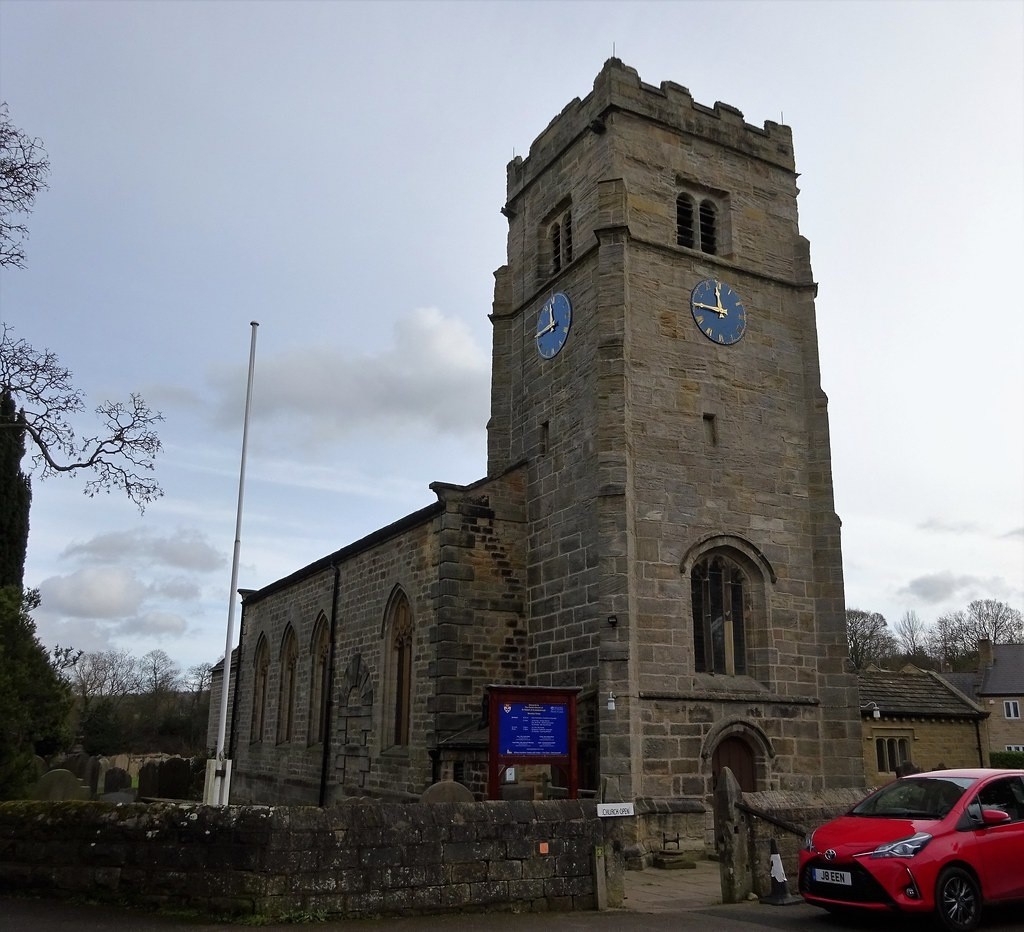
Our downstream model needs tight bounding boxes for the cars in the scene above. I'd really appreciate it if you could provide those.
[798,768,1024,932]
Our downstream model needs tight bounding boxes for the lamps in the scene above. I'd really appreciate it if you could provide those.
[860,701,881,718]
[607,691,616,711]
[608,615,618,627]
[703,410,717,420]
[984,698,995,706]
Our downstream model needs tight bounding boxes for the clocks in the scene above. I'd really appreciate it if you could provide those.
[530,293,572,361]
[690,276,748,348]
